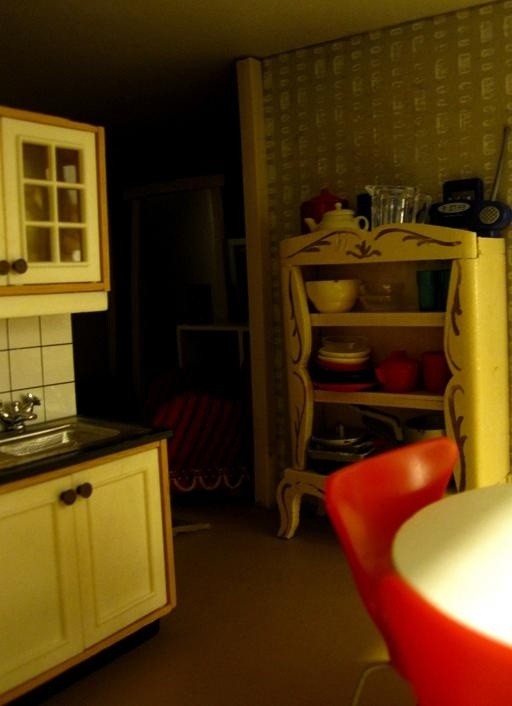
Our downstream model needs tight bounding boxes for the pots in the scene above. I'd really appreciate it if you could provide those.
[404,412,446,443]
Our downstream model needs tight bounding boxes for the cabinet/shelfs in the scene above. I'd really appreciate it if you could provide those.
[270,220,506,545]
[2,437,177,704]
[2,105,110,296]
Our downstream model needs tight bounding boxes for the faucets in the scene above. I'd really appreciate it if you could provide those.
[0,393,40,431]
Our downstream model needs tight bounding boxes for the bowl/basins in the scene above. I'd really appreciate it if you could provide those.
[359,282,411,311]
[317,342,374,373]
[305,278,361,313]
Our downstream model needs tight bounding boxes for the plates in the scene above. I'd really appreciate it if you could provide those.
[313,431,363,447]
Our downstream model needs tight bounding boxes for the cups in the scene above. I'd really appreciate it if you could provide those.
[362,182,432,229]
[421,351,448,393]
[414,269,446,312]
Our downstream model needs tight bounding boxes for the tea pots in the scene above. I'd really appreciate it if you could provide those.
[304,200,370,234]
[374,348,418,394]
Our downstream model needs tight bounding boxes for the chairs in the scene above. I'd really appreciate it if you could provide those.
[317,437,511,704]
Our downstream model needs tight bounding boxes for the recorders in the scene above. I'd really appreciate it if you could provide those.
[428,178,512,237]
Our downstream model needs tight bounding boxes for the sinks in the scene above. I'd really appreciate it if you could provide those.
[0,423,119,457]
